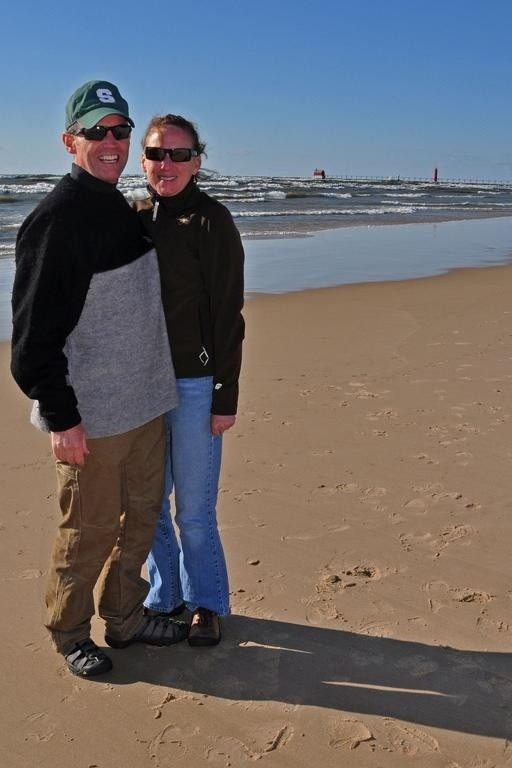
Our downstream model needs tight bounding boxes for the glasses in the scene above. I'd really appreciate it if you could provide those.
[80,125,132,140]
[144,146,198,163]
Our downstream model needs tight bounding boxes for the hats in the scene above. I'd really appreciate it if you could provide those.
[66,79,136,130]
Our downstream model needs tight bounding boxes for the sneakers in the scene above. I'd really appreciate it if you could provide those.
[62,637,112,676]
[190,608,221,646]
[142,601,185,618]
[106,616,191,649]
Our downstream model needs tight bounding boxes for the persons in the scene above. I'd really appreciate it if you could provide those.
[9,79,193,680]
[124,113,246,648]
